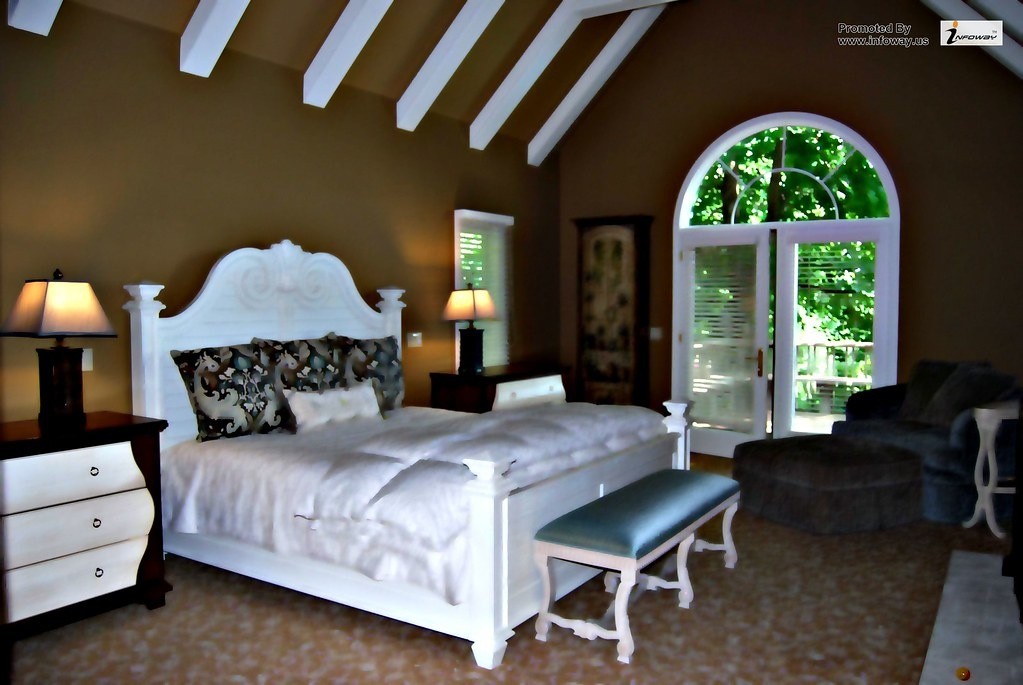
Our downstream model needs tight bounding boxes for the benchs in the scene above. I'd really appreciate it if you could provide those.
[534,468,740,666]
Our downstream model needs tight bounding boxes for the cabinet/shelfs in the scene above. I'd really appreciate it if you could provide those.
[567,213,656,414]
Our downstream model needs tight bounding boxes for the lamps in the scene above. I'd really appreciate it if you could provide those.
[437,284,499,376]
[1,268,120,436]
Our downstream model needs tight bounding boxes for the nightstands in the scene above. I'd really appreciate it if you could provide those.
[0,409,172,632]
[428,359,571,415]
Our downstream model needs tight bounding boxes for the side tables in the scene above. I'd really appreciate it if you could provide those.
[960,399,1021,539]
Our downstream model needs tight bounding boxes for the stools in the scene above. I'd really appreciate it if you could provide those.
[730,434,923,536]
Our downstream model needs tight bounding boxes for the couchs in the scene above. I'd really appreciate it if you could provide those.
[830,356,1023,524]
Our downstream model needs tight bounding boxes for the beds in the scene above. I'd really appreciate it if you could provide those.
[141,399,697,672]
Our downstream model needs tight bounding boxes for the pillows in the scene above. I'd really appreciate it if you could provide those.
[170,342,295,442]
[332,333,406,414]
[250,332,336,426]
[280,379,386,436]
